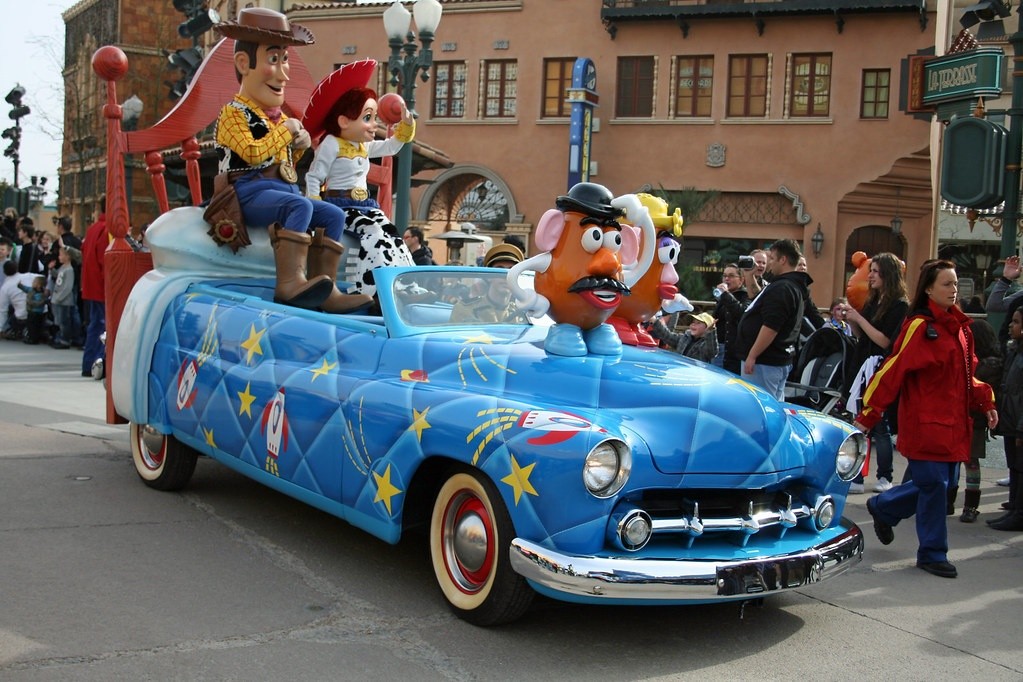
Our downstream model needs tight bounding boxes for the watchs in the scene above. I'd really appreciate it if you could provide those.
[841,309,848,322]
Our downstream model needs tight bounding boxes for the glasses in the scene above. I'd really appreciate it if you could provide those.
[721,274,743,279]
[403,235,416,239]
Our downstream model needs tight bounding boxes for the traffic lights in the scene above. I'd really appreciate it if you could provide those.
[936,119,1008,209]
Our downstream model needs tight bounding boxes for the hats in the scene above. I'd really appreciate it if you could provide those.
[484,243,524,267]
[691,312,715,328]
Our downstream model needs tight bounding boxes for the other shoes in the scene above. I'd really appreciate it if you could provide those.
[985,516,1005,525]
[0,330,86,351]
[82,372,92,377]
[991,521,1023,532]
[1001,502,1009,510]
[997,477,1010,486]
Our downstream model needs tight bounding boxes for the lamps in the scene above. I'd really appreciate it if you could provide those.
[890,186,903,235]
[812,223,825,259]
[162,0,220,101]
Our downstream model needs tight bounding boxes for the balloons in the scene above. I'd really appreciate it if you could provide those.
[847,251,906,314]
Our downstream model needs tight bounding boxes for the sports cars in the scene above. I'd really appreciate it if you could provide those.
[105,204,876,633]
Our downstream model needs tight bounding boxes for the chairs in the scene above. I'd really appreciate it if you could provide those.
[403,304,453,324]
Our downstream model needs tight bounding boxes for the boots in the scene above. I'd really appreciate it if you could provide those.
[946,485,958,515]
[960,488,982,522]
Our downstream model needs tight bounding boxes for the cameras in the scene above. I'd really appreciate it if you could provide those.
[738,255,754,269]
[838,303,847,315]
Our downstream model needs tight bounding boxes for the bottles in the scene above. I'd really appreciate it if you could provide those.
[713,283,728,297]
[655,310,662,320]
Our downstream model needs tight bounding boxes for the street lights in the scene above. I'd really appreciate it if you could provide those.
[376,0,443,236]
[118,95,144,222]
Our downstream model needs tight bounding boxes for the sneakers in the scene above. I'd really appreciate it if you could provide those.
[866,498,894,545]
[916,558,957,578]
[848,482,864,494]
[872,477,893,492]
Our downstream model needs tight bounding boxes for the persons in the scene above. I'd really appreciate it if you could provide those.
[211,7,374,314]
[0,193,151,381]
[402,225,530,326]
[301,58,436,317]
[642,241,1023,532]
[852,258,998,577]
